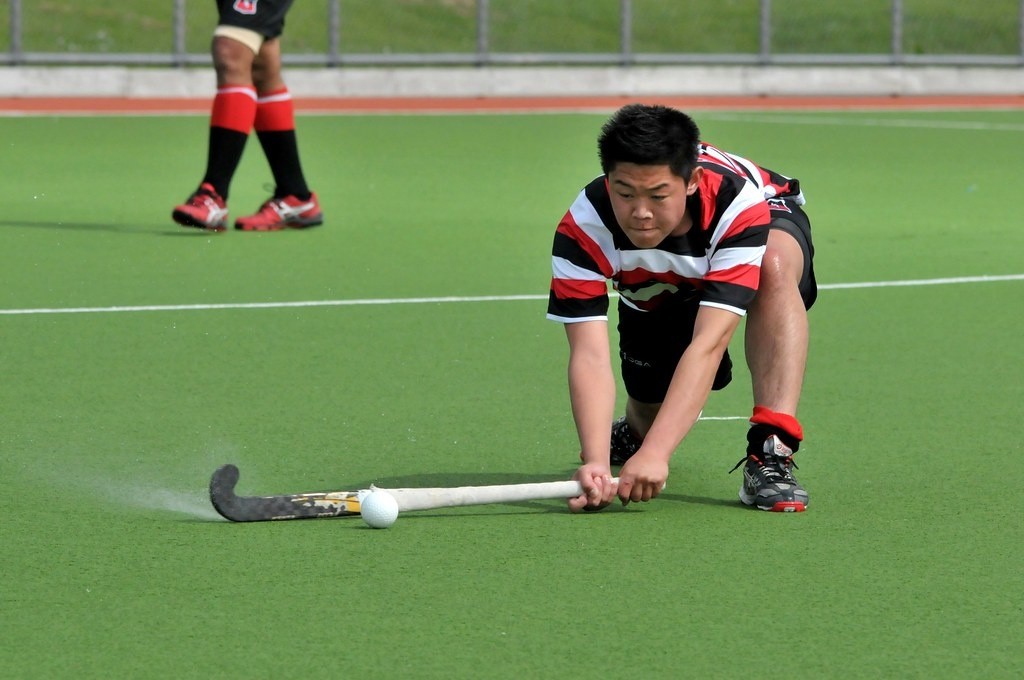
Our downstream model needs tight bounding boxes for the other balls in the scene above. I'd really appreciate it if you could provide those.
[361,491,398,529]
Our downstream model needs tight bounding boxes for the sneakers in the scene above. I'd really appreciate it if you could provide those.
[729,434,810,512]
[580,416,644,466]
[234,192,324,231]
[172,182,230,231]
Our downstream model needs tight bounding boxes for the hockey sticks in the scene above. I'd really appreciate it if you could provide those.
[207,461,666,525]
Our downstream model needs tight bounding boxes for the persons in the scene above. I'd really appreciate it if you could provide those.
[545,101,819,511]
[171,0,321,236]
